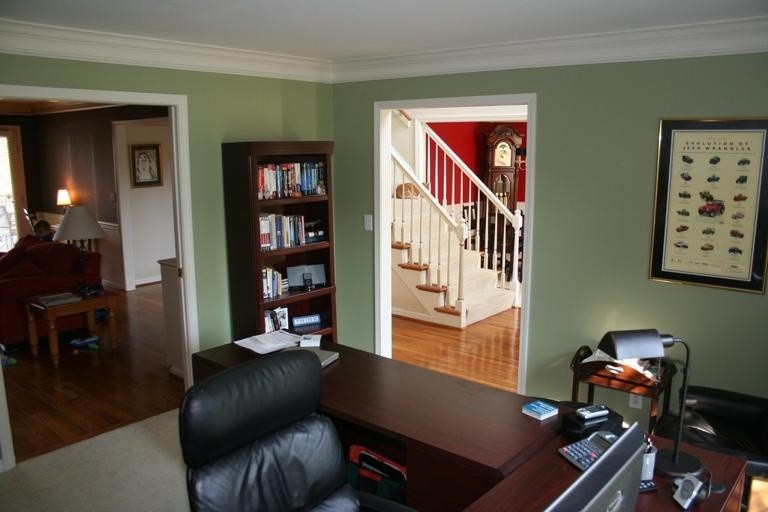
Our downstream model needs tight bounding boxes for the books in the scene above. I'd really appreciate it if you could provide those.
[257,161,327,336]
[520,398,559,421]
[38,292,82,307]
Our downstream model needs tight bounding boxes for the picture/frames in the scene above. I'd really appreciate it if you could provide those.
[128,143,164,189]
[647,114,767,299]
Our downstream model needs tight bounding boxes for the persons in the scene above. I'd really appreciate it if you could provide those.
[135,152,157,182]
[32,219,56,243]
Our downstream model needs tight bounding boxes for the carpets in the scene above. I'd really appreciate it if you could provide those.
[1,408,199,511]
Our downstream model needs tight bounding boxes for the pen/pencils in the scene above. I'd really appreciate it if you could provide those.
[643,428,655,453]
[32,304,45,309]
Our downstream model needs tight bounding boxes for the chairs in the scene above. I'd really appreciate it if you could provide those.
[177,347,364,512]
[461,200,523,284]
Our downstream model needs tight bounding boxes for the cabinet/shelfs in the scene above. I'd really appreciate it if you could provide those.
[191,329,576,511]
[221,140,339,344]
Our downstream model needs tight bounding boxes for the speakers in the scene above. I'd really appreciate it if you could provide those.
[672,472,707,510]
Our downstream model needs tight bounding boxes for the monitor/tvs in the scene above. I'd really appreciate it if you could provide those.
[542,421,644,512]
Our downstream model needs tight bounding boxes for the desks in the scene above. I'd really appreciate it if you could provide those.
[461,423,748,512]
[571,356,676,435]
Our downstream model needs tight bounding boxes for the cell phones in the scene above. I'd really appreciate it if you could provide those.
[576,404,609,420]
[582,417,608,426]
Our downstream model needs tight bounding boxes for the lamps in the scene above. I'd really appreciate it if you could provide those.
[56,189,74,212]
[598,329,705,479]
[51,206,106,296]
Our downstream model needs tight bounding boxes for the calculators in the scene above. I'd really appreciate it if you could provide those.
[557,430,619,471]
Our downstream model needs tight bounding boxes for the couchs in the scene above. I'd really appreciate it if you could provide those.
[1,234,102,354]
[655,385,767,512]
